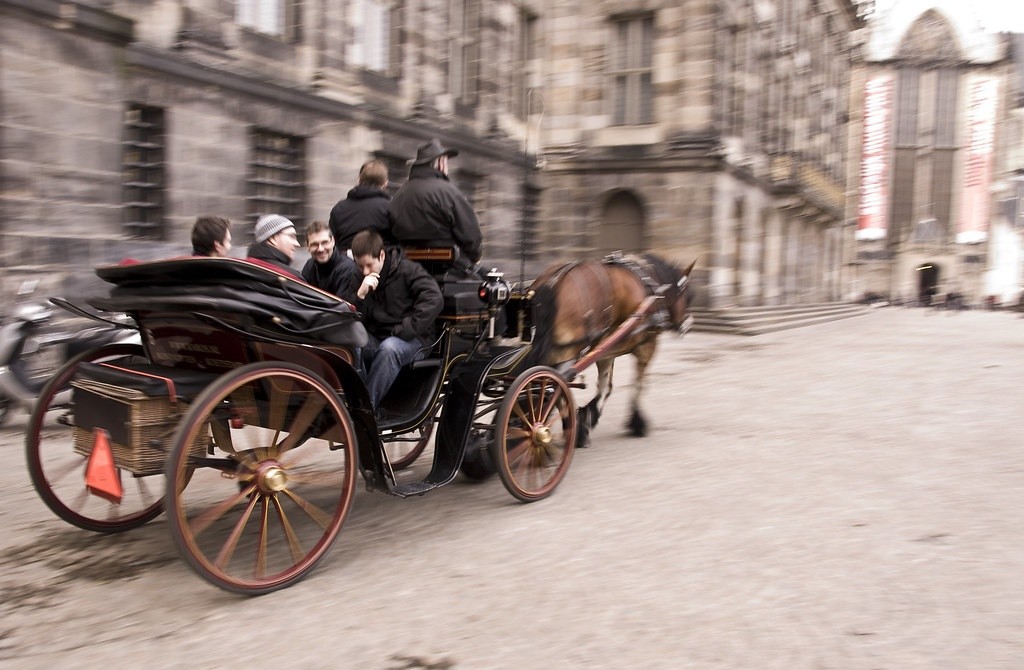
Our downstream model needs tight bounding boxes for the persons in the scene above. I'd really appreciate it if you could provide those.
[189,214,233,257]
[298,219,360,300]
[328,158,393,252]
[345,230,444,412]
[244,212,305,279]
[389,136,484,268]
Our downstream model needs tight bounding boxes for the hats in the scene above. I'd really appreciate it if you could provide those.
[253,213,296,244]
[405,139,459,165]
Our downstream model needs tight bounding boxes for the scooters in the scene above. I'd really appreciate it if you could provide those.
[0,293,143,429]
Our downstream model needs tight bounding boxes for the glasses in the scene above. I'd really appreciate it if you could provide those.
[281,231,298,240]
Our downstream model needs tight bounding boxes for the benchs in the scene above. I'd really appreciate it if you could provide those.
[406,320,450,368]
[396,241,491,320]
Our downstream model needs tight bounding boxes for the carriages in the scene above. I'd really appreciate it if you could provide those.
[24,245,696,595]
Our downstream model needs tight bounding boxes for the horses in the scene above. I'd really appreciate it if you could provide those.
[521,252,699,448]
